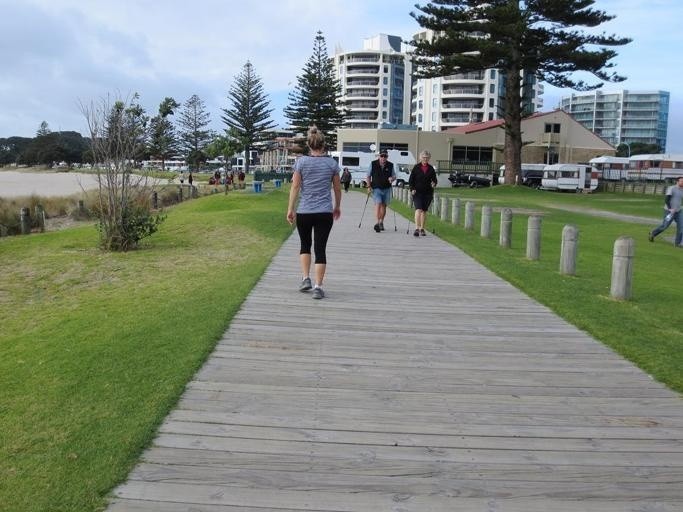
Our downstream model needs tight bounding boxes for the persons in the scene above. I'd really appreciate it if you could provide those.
[340,168,352,193]
[286,125,342,299]
[407,149,437,237]
[365,149,397,233]
[647,177,683,248]
[214,169,221,186]
[238,170,245,183]
[188,173,193,184]
[178,171,184,183]
[225,170,233,185]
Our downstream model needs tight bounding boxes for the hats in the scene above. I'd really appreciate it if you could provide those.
[380,150,387,154]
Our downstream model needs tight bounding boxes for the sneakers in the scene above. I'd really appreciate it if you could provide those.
[312,288,325,299]
[649,232,654,242]
[413,229,425,236]
[374,222,384,233]
[298,278,312,291]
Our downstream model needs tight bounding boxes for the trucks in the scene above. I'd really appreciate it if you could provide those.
[333,152,412,189]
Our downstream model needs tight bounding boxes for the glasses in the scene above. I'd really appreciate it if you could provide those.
[381,154,388,158]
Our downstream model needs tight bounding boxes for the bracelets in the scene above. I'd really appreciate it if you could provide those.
[367,184,370,188]
